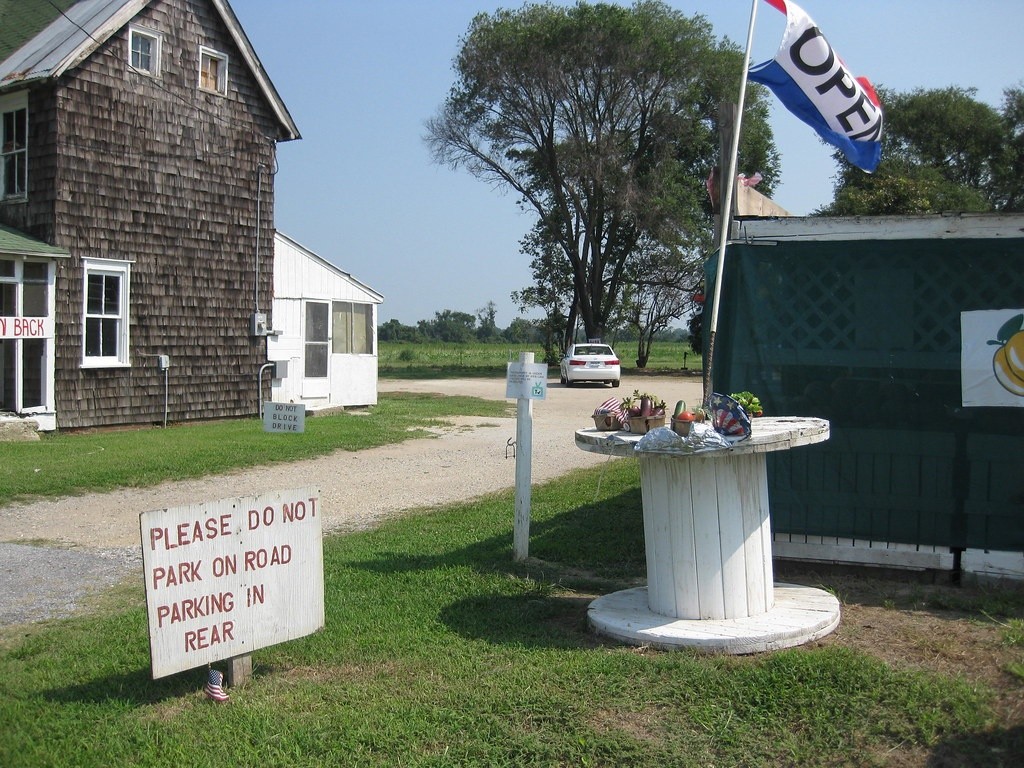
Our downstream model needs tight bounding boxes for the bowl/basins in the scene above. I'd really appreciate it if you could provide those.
[670,418,693,438]
[628,415,665,435]
[592,414,622,432]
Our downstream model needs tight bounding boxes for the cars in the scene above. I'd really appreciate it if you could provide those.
[560,343,621,387]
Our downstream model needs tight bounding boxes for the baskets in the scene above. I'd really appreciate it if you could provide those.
[591,413,623,430]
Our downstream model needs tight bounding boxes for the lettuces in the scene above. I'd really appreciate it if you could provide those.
[731,390,763,416]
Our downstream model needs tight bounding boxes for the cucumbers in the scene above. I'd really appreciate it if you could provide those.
[674,400,686,419]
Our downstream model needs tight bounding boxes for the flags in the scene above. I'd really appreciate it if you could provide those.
[747,0,884,175]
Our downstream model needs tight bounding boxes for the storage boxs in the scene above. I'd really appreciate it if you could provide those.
[629,415,665,435]
[670,419,691,437]
[592,414,622,431]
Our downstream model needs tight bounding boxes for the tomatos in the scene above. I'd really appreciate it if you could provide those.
[678,411,694,421]
